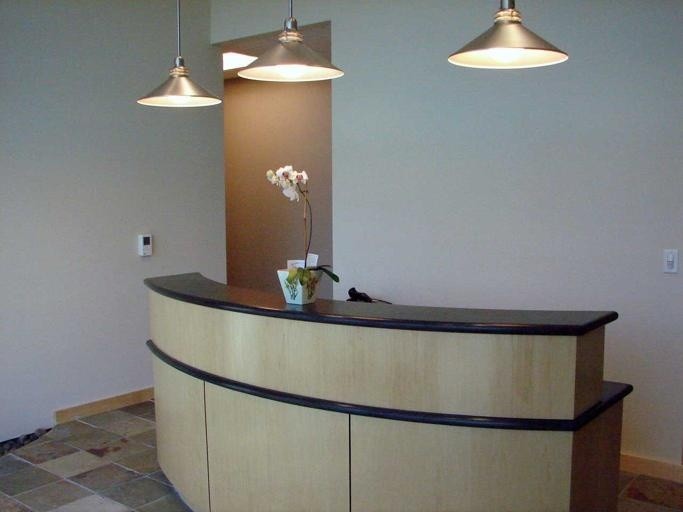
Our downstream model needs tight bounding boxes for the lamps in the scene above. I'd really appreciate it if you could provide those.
[136,0,344,109]
[448,0,569,72]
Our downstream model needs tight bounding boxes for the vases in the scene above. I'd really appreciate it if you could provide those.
[276,269,324,305]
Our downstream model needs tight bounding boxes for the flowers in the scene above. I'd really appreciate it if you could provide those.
[266,166,339,286]
